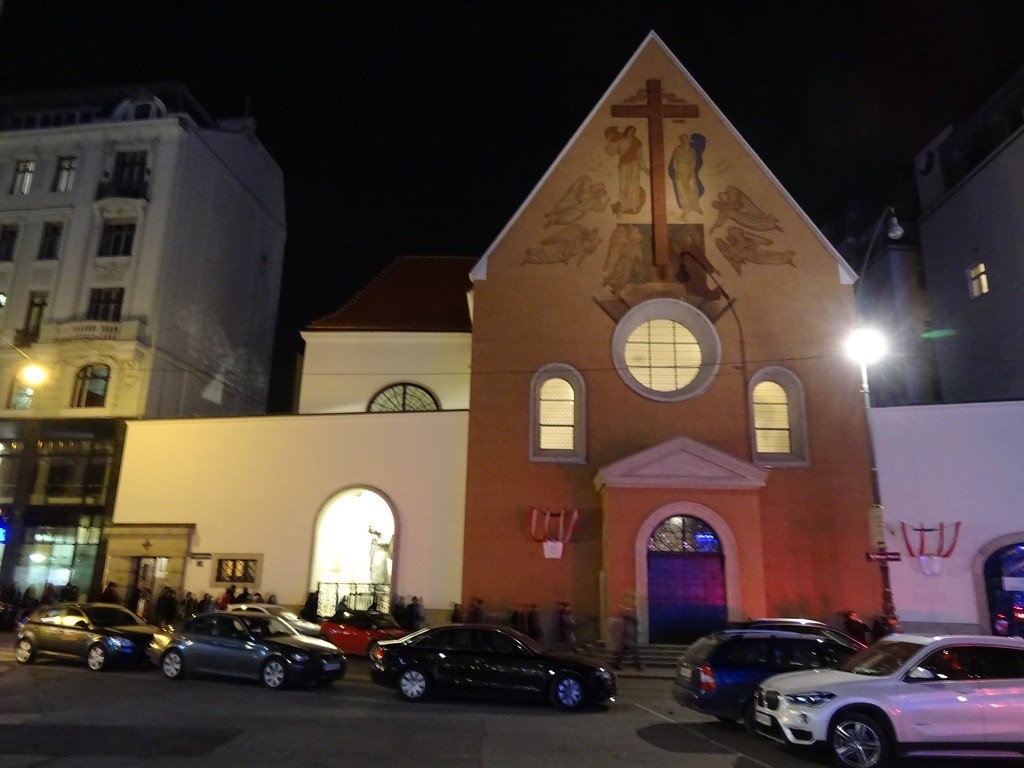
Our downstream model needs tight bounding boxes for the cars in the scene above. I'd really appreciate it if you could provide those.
[140,611,345,695]
[369,627,621,715]
[725,614,868,660]
[309,604,403,657]
[670,629,861,735]
[228,604,321,643]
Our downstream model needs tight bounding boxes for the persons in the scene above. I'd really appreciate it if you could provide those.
[0,565,912,673]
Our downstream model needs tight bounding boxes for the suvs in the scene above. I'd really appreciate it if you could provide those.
[15,603,156,676]
[754,630,1021,765]
